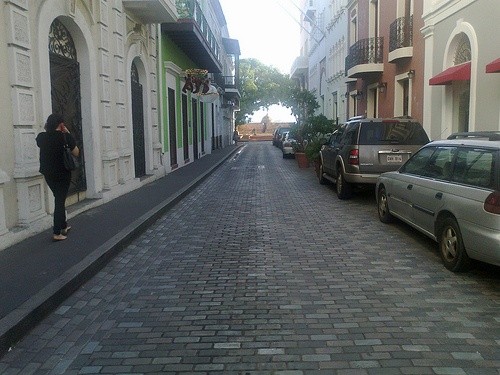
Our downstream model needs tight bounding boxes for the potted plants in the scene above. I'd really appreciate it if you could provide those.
[282,87,337,168]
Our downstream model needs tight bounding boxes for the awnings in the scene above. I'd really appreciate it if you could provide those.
[486,58,500,73]
[429,62,471,86]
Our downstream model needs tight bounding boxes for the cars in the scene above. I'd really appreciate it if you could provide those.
[282,131,311,158]
[273,126,294,149]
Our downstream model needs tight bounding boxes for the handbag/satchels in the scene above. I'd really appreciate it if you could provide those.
[62,132,80,171]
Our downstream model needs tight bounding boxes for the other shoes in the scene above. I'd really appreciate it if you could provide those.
[54,234,67,240]
[63,226,72,234]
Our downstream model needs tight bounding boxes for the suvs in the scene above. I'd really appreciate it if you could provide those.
[375,131,500,272]
[318,115,430,198]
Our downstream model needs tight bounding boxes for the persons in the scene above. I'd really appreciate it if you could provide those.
[35,113,79,240]
